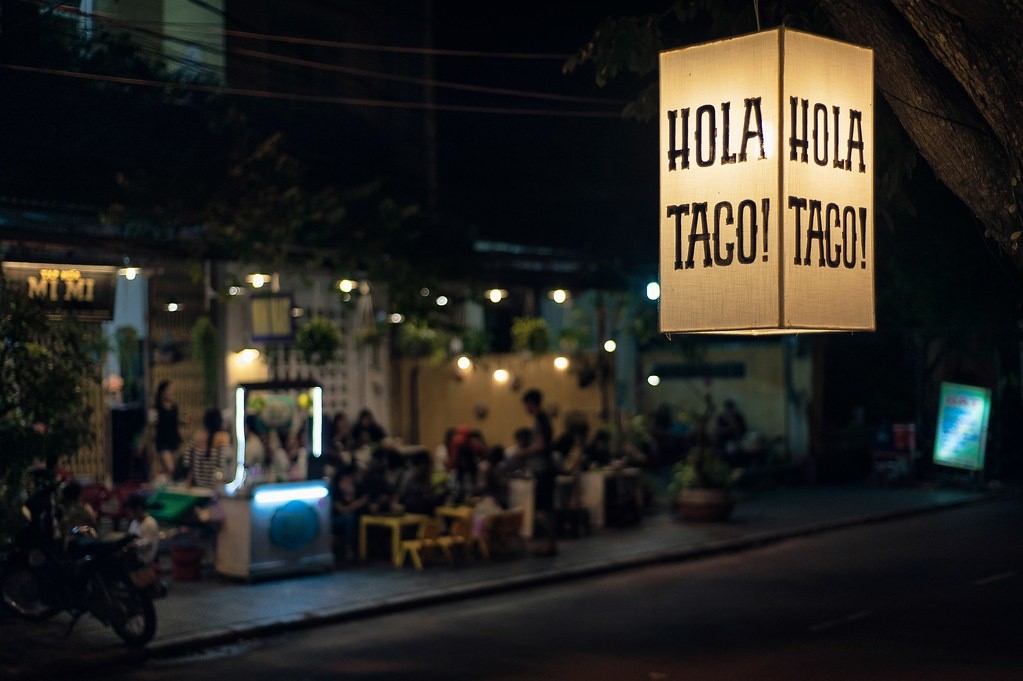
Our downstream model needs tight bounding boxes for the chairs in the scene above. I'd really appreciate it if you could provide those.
[63,431,680,579]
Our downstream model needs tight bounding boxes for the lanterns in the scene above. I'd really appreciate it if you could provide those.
[658,1,875,338]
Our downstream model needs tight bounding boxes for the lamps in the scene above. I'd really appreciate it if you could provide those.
[68,264,669,392]
[656,20,881,340]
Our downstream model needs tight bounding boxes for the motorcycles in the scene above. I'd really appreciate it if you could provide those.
[0,454,162,646]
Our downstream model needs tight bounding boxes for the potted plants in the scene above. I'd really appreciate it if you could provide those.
[672,383,726,524]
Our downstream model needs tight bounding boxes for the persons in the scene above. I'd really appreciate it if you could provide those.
[5,467,163,580]
[137,377,757,559]
